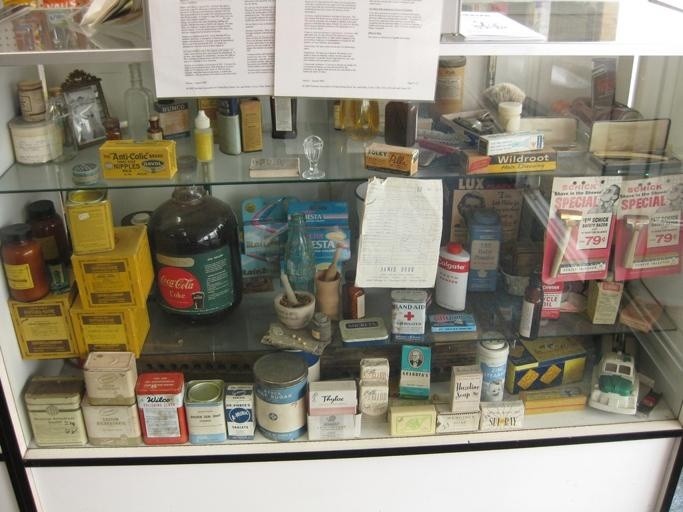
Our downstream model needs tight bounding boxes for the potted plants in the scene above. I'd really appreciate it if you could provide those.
[59,69,109,151]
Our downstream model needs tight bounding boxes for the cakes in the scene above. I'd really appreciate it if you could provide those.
[591,58,617,108]
[19,80,46,123]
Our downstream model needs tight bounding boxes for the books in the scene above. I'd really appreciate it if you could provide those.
[69,295,151,359]
[7,287,79,359]
[70,224,155,309]
[506,338,587,395]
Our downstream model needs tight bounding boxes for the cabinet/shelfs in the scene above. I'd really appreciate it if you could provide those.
[146,116,164,140]
[475,331,509,403]
[498,101,522,134]
[518,269,542,341]
[148,155,243,327]
[435,210,502,312]
[192,109,214,163]
[311,310,331,342]
[124,63,156,140]
[45,87,78,164]
[342,270,366,319]
[0,199,69,303]
[286,210,316,294]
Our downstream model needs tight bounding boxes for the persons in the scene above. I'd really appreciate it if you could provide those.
[586,184,619,215]
[656,182,683,212]
[457,191,484,231]
[409,350,421,366]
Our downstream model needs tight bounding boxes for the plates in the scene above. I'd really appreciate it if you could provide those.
[0,0,683,512]
